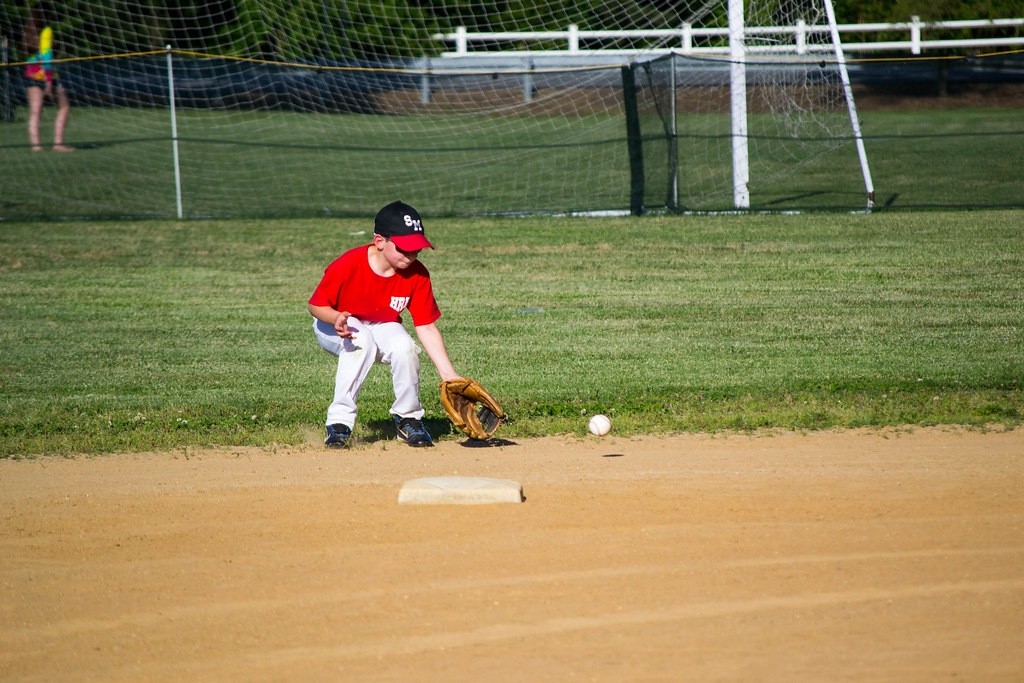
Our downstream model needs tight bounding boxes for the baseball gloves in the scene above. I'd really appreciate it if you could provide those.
[439,377,507,441]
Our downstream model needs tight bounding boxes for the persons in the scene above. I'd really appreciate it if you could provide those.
[307,199,465,446]
[24,3,76,155]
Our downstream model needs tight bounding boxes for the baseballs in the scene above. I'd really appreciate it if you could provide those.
[589,414,611,437]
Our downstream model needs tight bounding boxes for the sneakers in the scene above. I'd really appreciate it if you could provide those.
[323,423,352,449]
[392,414,433,447]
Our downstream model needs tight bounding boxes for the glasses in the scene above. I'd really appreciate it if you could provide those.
[374,232,423,254]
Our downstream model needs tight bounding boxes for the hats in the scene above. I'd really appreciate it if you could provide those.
[373,201,434,253]
